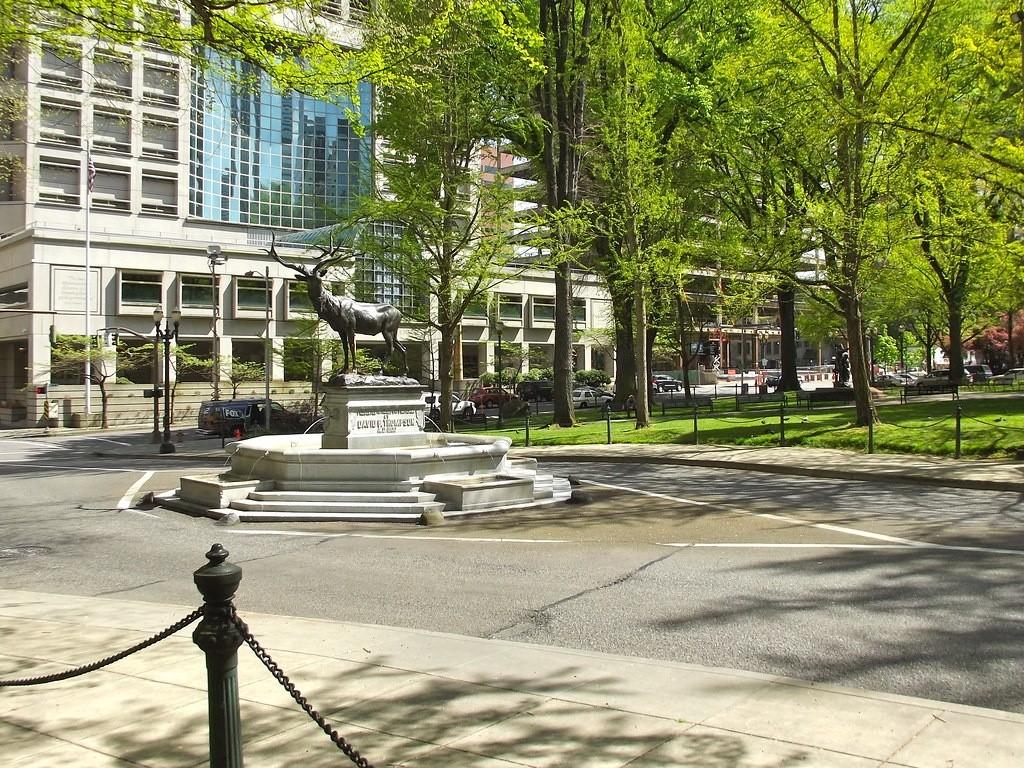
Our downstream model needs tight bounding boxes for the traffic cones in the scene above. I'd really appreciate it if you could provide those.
[236,428,242,439]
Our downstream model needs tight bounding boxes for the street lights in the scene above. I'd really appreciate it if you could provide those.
[898,324,906,371]
[152,304,182,453]
[495,318,504,430]
[868,319,874,383]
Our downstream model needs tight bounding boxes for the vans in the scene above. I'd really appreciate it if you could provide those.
[199,395,285,437]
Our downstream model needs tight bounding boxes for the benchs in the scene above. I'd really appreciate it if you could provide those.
[807,391,855,411]
[870,380,893,394]
[991,377,1015,392]
[900,384,960,405]
[795,391,823,408]
[662,396,715,416]
[601,403,652,421]
[969,378,991,392]
[958,379,971,391]
[433,414,488,432]
[735,392,789,411]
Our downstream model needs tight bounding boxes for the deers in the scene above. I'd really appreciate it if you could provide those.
[257,229,409,377]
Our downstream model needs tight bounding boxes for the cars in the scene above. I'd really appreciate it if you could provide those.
[420,390,477,421]
[470,386,520,409]
[570,374,684,408]
[514,378,555,401]
[871,363,1024,387]
[767,374,804,387]
